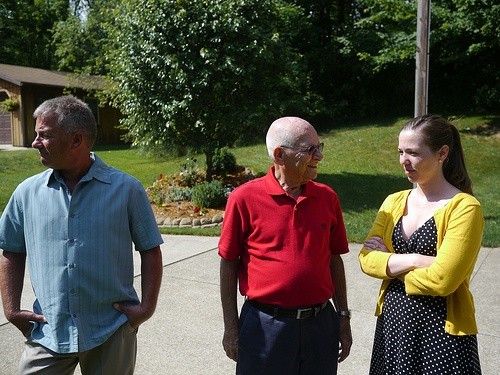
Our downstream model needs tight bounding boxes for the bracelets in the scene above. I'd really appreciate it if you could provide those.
[336,310,352,319]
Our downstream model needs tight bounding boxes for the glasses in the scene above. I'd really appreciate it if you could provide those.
[280,143,324,155]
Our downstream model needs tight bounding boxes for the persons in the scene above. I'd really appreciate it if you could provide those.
[0,94,165,375]
[218,117,353,375]
[357,114,485,375]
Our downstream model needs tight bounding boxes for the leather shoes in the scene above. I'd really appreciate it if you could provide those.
[249,299,328,320]
